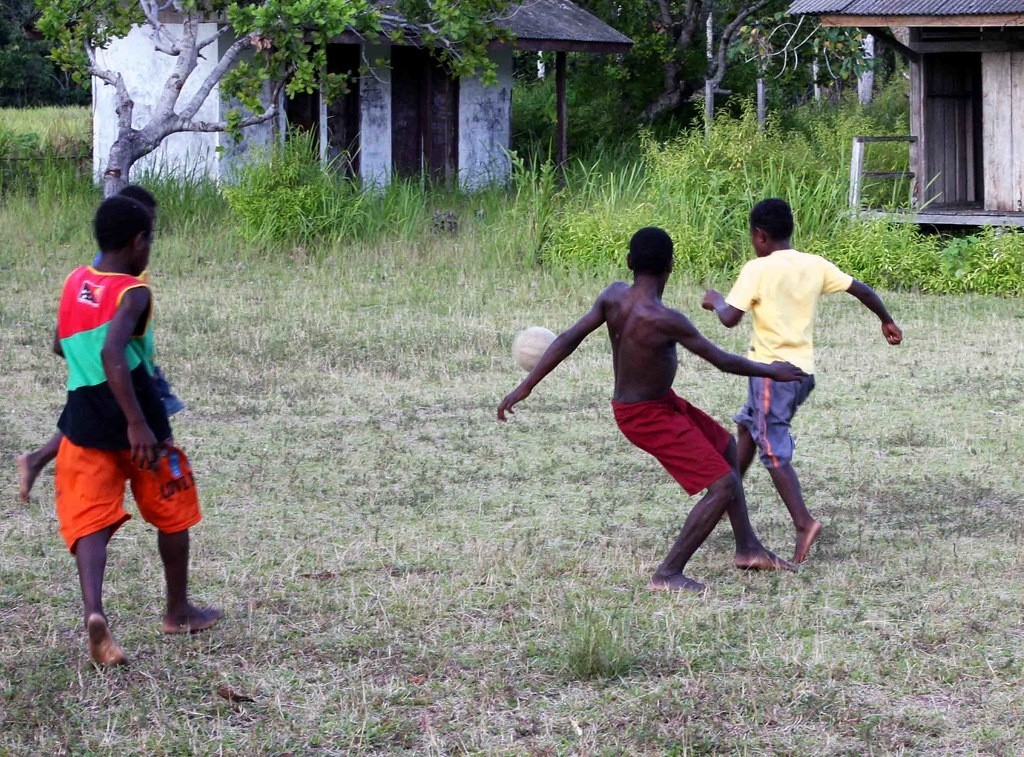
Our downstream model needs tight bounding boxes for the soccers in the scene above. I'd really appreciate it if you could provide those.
[511,325,559,374]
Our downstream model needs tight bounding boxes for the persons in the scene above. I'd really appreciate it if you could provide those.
[58,195,224,669]
[701,199,903,564]
[19,186,187,502]
[497,227,808,589]
